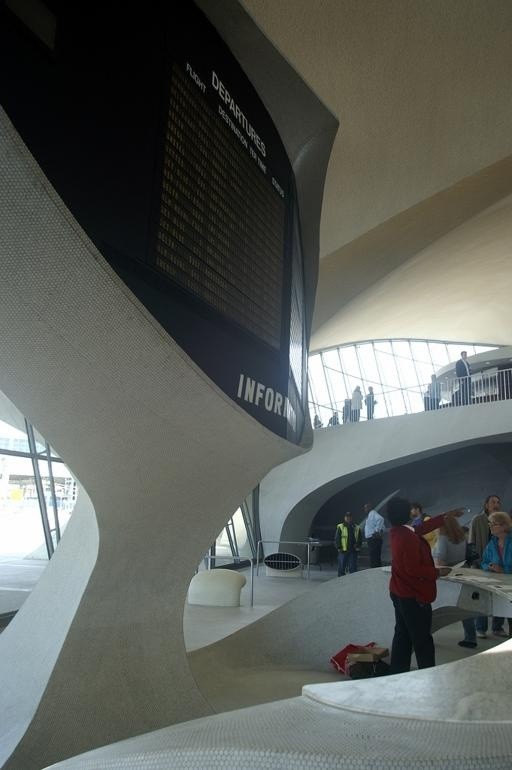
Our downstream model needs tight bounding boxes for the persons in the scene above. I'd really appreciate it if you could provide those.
[327,412,339,427]
[313,415,323,429]
[334,511,363,577]
[364,502,385,567]
[479,511,512,639]
[429,374,441,410]
[342,399,351,424]
[424,384,431,412]
[455,351,471,406]
[351,386,363,423]
[364,386,377,420]
[470,493,509,638]
[409,502,439,554]
[386,496,467,674]
[431,514,477,649]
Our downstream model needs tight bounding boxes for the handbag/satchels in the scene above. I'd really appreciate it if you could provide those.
[329,642,374,672]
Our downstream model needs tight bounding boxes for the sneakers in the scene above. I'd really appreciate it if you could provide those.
[458,630,509,647]
